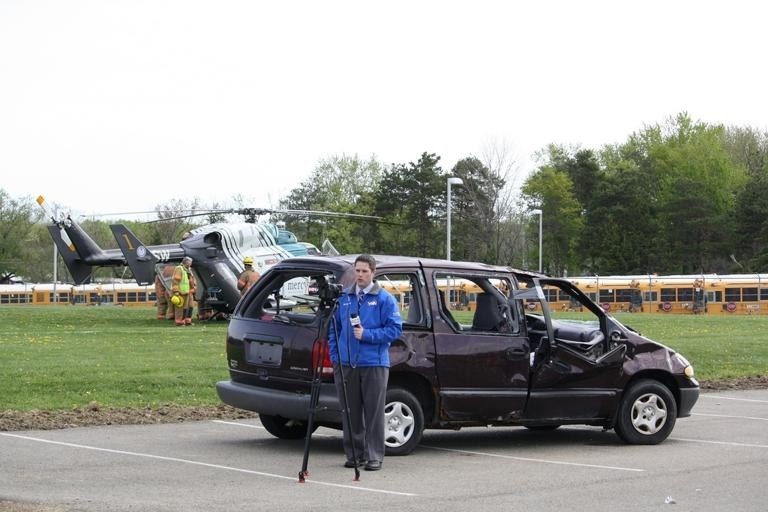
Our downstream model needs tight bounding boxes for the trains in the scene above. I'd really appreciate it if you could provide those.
[0,272,768,317]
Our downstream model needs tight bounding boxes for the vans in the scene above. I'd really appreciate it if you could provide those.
[214,252,702,457]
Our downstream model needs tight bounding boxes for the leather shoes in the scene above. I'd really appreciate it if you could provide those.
[366,461,382,470]
[345,458,366,467]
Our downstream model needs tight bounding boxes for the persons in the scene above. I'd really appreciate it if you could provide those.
[238,256,262,300]
[329,255,402,471]
[155,255,213,325]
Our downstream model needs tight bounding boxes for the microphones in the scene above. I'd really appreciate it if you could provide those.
[349,312,362,346]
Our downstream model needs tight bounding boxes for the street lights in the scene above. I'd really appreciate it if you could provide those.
[530,208,544,274]
[445,176,465,311]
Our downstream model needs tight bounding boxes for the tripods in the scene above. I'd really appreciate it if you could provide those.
[298,299,361,484]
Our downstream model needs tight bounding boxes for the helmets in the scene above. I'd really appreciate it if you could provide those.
[244,256,253,264]
[171,295,185,307]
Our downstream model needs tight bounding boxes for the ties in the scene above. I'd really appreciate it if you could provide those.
[357,289,364,314]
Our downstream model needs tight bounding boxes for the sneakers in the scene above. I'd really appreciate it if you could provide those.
[175,323,195,326]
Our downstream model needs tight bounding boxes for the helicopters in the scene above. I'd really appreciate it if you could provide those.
[36,193,406,323]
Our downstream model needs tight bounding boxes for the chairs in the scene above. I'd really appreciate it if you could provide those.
[405,288,511,332]
